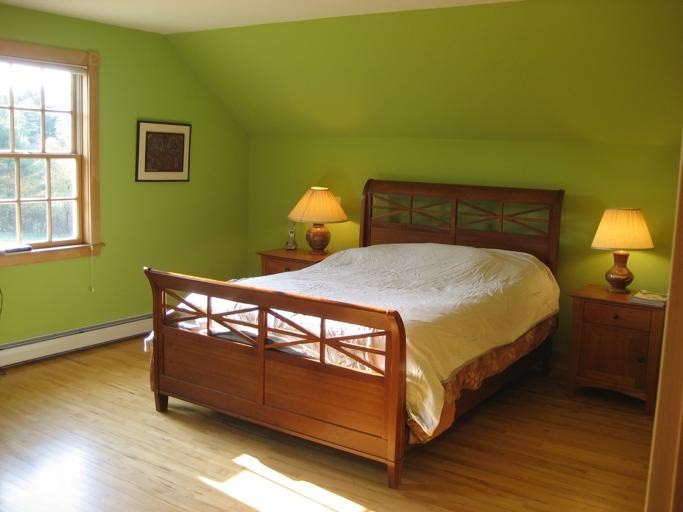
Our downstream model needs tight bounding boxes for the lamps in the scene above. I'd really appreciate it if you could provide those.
[590,206,656,294]
[287,186,349,250]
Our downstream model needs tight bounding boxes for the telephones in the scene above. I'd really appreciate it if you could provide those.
[285,222,297,249]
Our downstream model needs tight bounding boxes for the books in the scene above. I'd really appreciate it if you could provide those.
[630,288,667,307]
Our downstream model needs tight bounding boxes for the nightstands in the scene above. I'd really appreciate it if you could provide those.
[253,246,332,276]
[567,283,666,421]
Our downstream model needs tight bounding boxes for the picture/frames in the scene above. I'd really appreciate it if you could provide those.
[134,120,192,181]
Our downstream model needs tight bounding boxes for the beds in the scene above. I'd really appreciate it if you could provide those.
[143,178,565,490]
